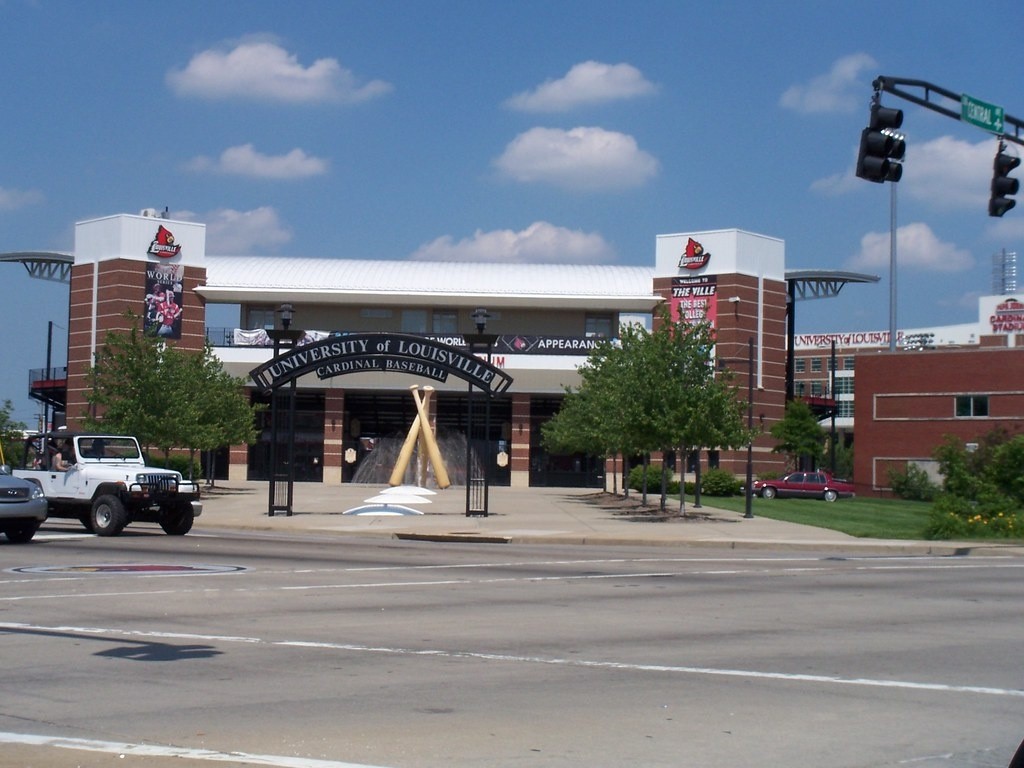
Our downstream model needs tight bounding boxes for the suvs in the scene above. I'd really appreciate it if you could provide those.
[9,431,202,538]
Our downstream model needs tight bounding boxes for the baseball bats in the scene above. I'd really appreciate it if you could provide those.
[388,384,451,490]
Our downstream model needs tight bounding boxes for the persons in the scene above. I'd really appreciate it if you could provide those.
[83,439,110,457]
[50,453,69,472]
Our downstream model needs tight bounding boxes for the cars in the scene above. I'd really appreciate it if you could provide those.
[0,465,49,544]
[752,470,856,502]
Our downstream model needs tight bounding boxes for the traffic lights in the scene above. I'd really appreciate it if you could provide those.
[989,152,1022,217]
[856,103,906,184]
